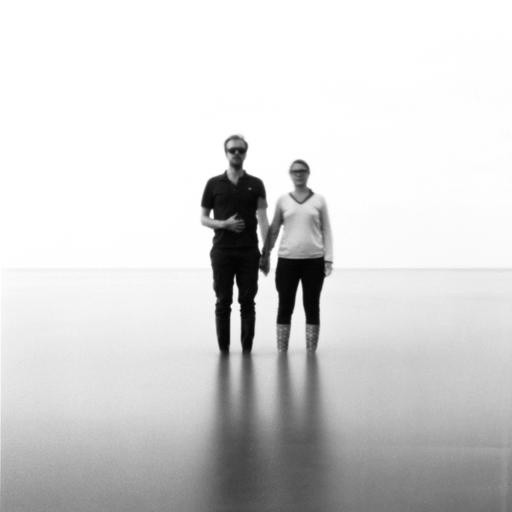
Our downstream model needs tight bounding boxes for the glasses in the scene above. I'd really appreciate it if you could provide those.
[227,147,246,154]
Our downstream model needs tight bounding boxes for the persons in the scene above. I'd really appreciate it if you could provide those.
[201,135,272,352]
[261,159,332,358]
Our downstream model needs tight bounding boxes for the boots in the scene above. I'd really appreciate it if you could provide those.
[277,324,290,349]
[306,324,319,349]
